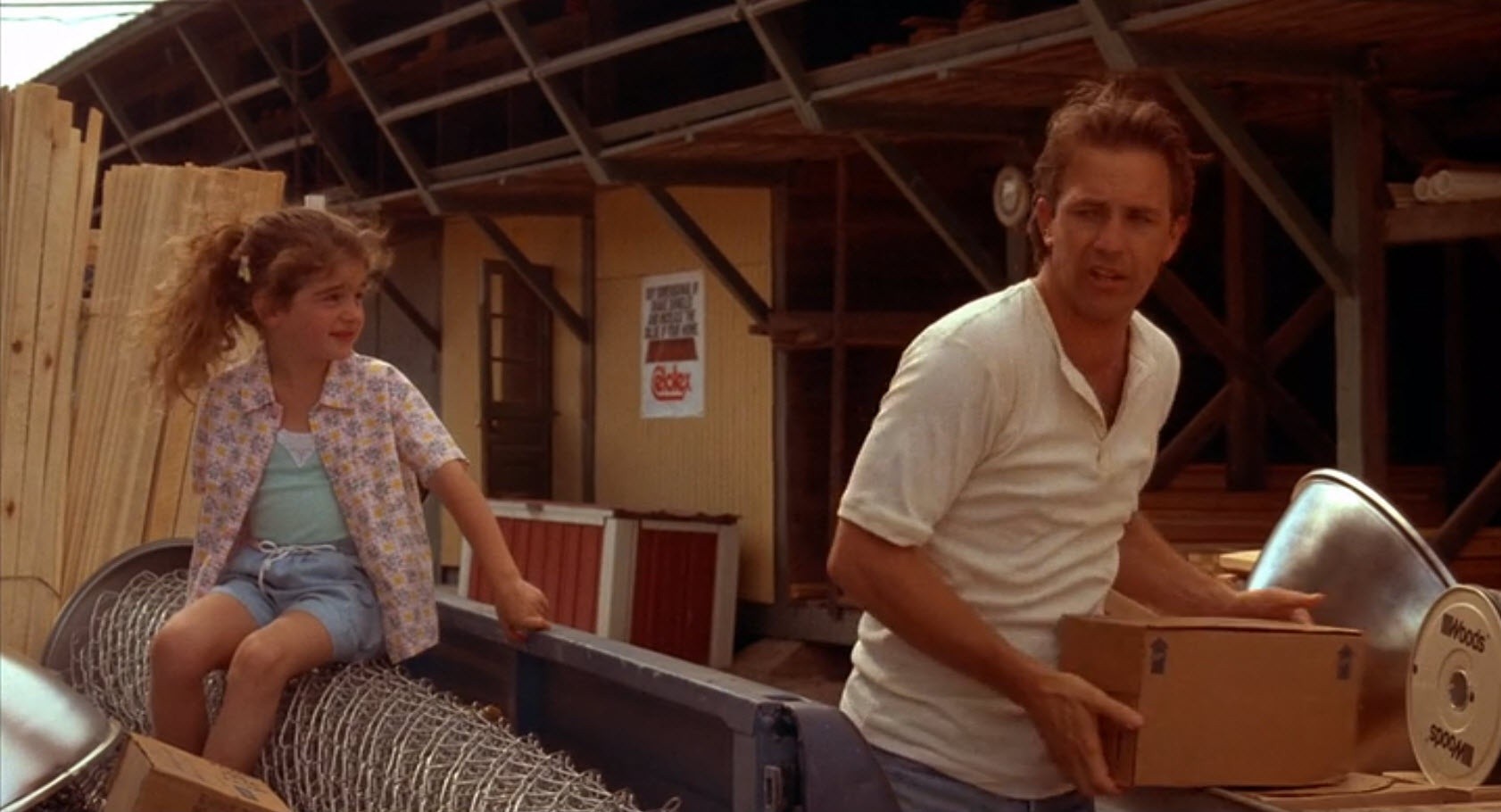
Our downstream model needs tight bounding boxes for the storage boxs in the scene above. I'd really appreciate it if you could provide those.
[105,732,290,812]
[1057,612,1364,789]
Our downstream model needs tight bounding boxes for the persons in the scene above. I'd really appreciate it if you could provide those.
[825,75,1325,812]
[118,205,554,776]
